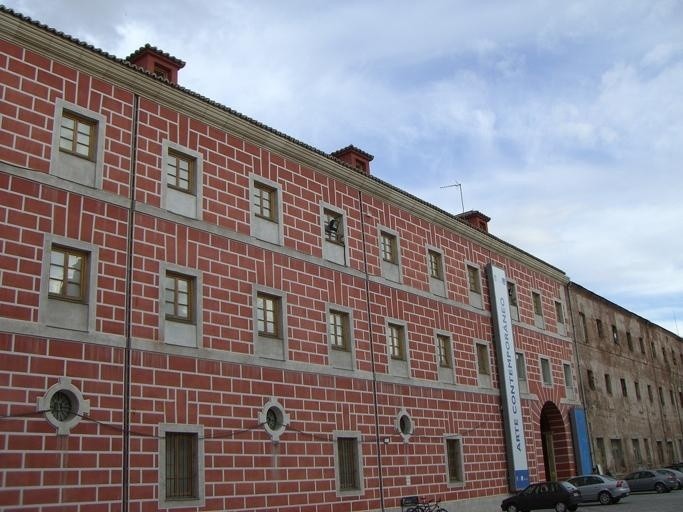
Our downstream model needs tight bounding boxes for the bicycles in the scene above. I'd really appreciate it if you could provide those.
[406,497,448,512]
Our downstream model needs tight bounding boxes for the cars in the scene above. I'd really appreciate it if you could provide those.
[566,474,631,505]
[622,463,683,493]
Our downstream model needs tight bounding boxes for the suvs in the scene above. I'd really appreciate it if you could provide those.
[500,480,582,512]
